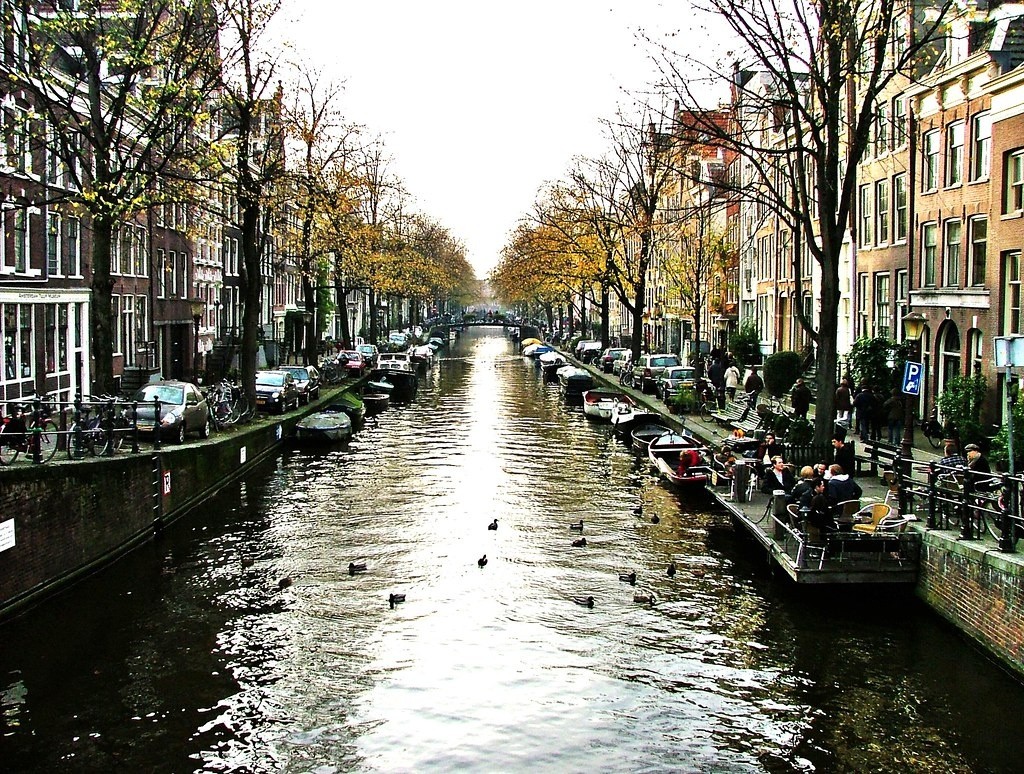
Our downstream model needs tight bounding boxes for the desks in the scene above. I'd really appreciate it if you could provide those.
[741,458,762,477]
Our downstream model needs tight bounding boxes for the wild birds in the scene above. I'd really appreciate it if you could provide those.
[237,519,296,611]
[596,442,612,477]
[478,555,488,568]
[633,504,644,514]
[633,595,656,603]
[574,596,594,605]
[651,513,660,525]
[570,520,584,530]
[573,537,587,547]
[389,593,406,603]
[628,451,645,486]
[488,519,499,530]
[689,564,708,578]
[667,563,675,577]
[346,562,368,572]
[618,572,636,582]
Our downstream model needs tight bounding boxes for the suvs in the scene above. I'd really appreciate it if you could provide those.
[277,364,322,404]
[631,354,682,394]
[354,344,379,367]
[256,371,299,415]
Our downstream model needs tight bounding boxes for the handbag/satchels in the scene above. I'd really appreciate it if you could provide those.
[737,376,740,384]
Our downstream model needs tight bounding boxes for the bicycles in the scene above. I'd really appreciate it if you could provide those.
[923,405,945,448]
[67,392,132,461]
[737,389,797,422]
[319,356,350,384]
[621,372,632,387]
[699,391,734,422]
[0,389,60,466]
[937,465,1024,549]
[200,378,256,433]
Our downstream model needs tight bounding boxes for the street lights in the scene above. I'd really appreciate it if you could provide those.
[299,310,313,367]
[190,297,206,380]
[398,310,403,333]
[641,313,649,355]
[378,310,385,345]
[902,312,929,500]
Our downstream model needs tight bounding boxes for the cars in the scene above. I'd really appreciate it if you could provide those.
[126,381,210,444]
[336,349,366,378]
[574,340,696,406]
[384,325,424,353]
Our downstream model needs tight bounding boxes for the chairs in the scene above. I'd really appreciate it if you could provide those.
[714,453,908,570]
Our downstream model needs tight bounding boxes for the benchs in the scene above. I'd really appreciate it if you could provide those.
[853,440,901,481]
[710,403,762,432]
[650,448,704,452]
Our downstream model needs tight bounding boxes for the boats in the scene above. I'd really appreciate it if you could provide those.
[522,337,593,388]
[368,353,419,391]
[296,377,396,443]
[582,390,731,488]
[408,331,447,360]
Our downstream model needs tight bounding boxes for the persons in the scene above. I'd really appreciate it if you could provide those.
[790,460,863,558]
[761,456,802,531]
[724,362,740,402]
[831,432,854,479]
[745,367,763,409]
[958,444,995,492]
[791,378,811,418]
[710,345,729,369]
[715,445,736,478]
[883,389,904,446]
[708,358,722,386]
[751,433,782,479]
[835,376,885,441]
[487,309,493,317]
[677,450,704,477]
[914,443,967,500]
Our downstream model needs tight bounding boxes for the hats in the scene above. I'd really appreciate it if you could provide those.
[889,387,901,396]
[965,443,979,451]
